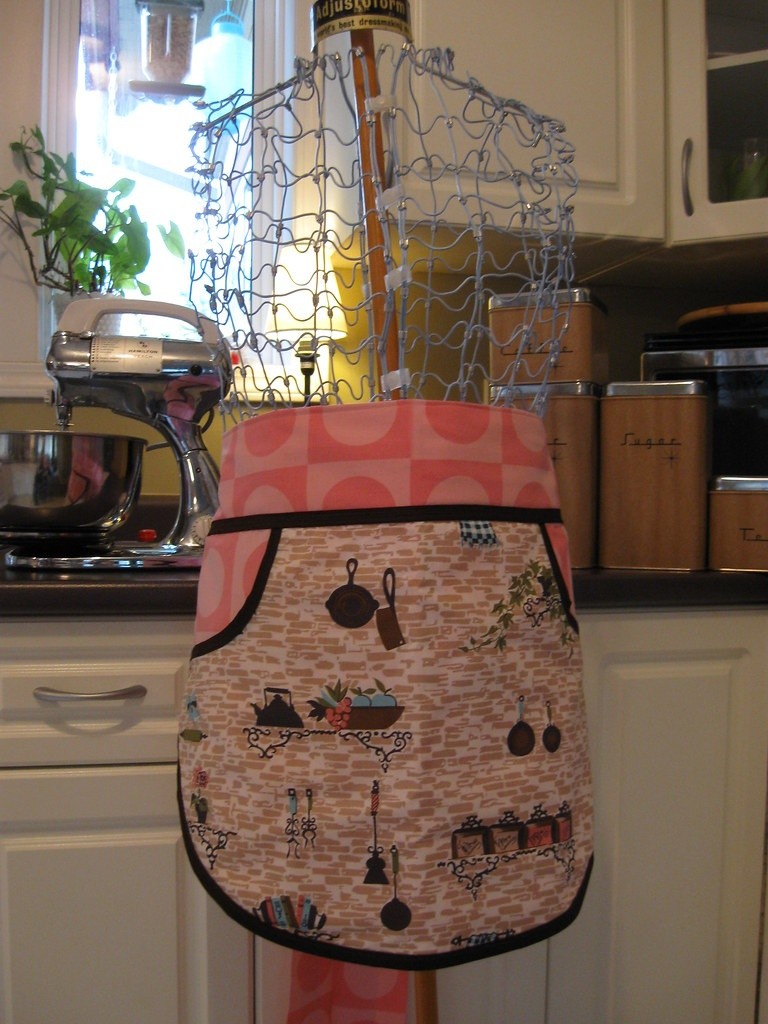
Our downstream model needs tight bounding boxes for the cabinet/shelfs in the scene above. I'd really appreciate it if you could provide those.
[300,0,768,288]
[0,604,768,1024]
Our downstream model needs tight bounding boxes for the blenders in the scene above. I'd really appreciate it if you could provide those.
[0,298,232,572]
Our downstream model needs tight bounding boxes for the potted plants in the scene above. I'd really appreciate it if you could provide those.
[1,123,186,334]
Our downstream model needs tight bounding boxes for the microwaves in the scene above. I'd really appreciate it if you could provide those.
[642,330,768,489]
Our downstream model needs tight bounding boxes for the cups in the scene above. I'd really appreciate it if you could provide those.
[742,139,766,169]
